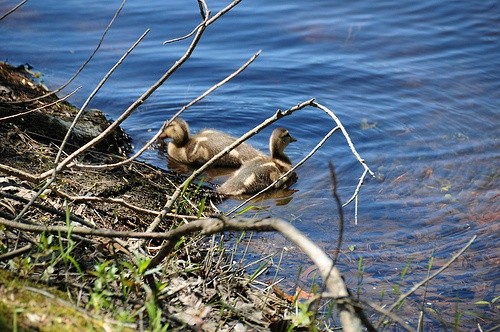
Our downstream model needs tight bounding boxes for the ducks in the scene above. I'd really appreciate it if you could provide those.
[159,118,265,166]
[215,127,298,196]
